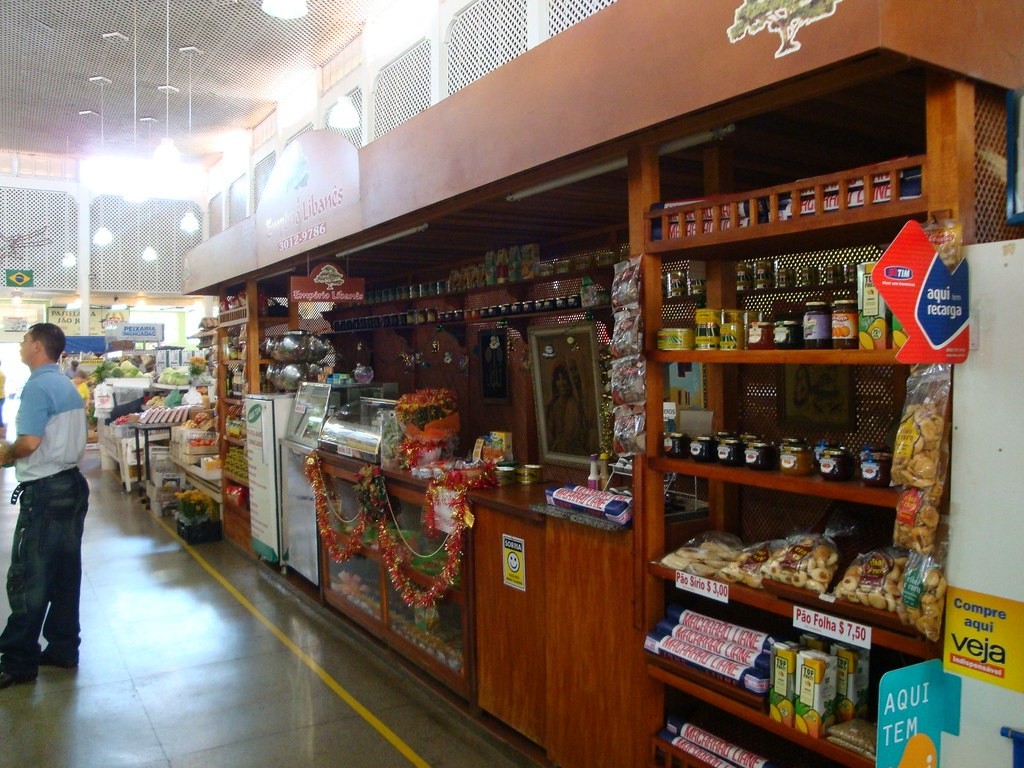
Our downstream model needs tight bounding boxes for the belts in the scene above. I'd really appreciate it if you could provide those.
[11,466,77,504]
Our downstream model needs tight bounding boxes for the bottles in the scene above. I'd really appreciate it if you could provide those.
[355,366,374,384]
[587,454,600,491]
[598,453,610,491]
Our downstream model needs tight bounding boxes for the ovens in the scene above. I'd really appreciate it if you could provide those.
[317,396,398,464]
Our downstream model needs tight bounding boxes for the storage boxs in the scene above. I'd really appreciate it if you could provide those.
[830,642,869,724]
[144,443,222,545]
[490,431,513,462]
[891,314,909,350]
[856,262,892,349]
[520,244,540,279]
[769,640,807,729]
[800,633,835,653]
[794,648,838,739]
[112,340,132,350]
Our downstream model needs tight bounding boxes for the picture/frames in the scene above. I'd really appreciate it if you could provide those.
[524,321,608,469]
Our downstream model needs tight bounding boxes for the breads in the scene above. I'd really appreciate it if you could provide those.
[661,540,945,644]
[891,402,945,554]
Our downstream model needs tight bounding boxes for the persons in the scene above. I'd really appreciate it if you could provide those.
[545,365,589,456]
[0,320,90,688]
[63,360,82,380]
[0,360,6,427]
[71,371,90,443]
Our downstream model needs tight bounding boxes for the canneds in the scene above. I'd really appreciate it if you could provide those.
[665,431,895,489]
[657,258,859,351]
[331,279,581,330]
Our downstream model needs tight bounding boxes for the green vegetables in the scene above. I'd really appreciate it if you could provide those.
[93,364,105,384]
[89,408,97,425]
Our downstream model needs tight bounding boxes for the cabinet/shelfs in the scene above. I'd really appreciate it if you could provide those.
[214,65,1024,768]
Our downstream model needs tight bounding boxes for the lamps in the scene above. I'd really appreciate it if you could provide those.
[62,0,359,314]
[11,288,24,304]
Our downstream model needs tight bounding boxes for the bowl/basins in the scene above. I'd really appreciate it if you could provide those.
[496,467,514,486]
[516,464,544,485]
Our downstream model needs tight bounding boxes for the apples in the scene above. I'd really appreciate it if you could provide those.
[185,436,216,446]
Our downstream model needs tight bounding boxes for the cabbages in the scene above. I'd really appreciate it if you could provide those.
[101,360,153,378]
[157,367,189,386]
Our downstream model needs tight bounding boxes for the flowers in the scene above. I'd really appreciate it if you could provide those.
[175,489,212,522]
[394,387,460,444]
[188,356,206,375]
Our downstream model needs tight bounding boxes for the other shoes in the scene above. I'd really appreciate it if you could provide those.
[0,671,37,689]
[38,651,78,669]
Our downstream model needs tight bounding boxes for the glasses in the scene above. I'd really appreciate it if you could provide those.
[73,364,78,367]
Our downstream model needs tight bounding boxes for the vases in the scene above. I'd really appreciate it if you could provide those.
[409,441,442,467]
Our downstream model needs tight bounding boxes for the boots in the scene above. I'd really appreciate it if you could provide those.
[0,427,6,439]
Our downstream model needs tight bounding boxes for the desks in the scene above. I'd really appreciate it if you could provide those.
[127,422,183,509]
